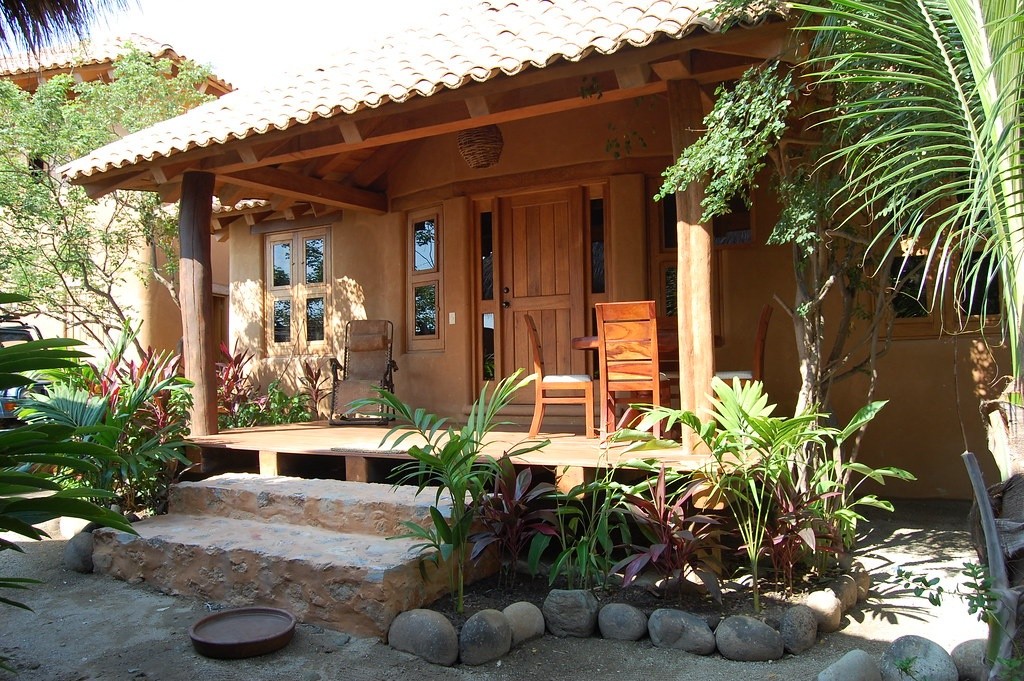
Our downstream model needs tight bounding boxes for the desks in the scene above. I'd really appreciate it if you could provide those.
[572,329,722,436]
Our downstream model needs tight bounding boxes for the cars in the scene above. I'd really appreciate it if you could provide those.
[0,326,63,424]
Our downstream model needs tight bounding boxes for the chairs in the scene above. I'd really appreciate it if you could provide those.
[714,306,775,404]
[522,316,593,439]
[593,301,679,447]
[328,319,398,426]
